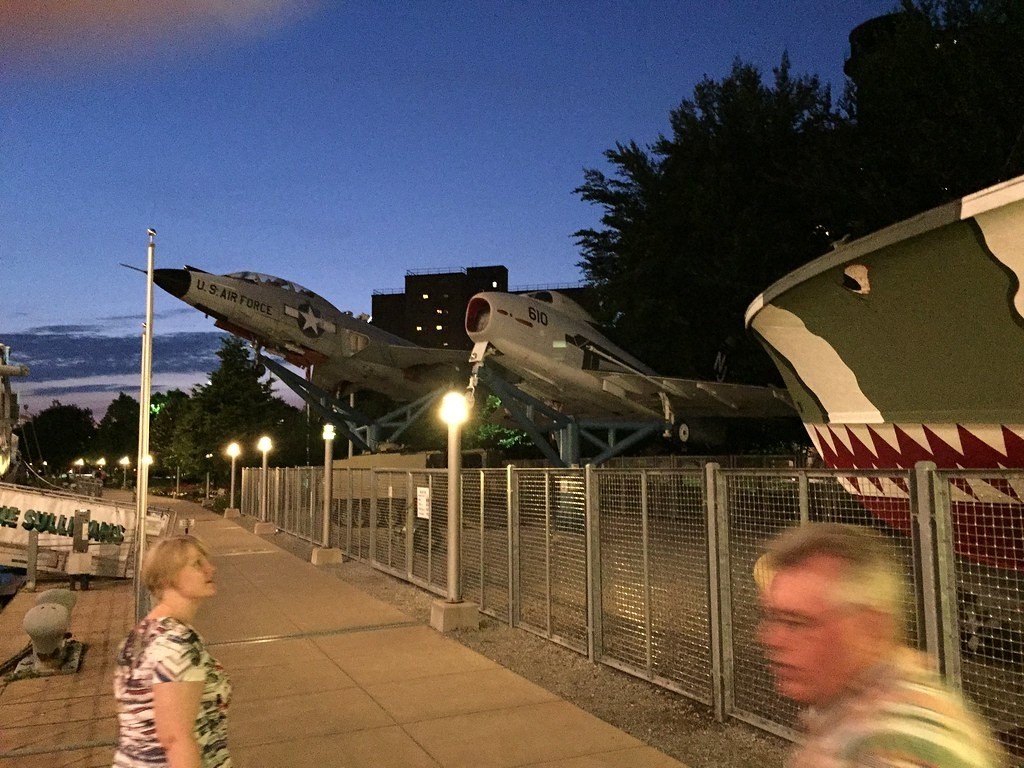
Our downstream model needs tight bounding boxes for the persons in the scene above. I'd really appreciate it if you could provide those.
[108,534,233,768]
[752,524,1006,767]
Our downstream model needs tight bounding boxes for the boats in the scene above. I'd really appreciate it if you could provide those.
[744,173,1023,577]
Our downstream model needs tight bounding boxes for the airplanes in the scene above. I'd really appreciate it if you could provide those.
[115,256,804,535]
